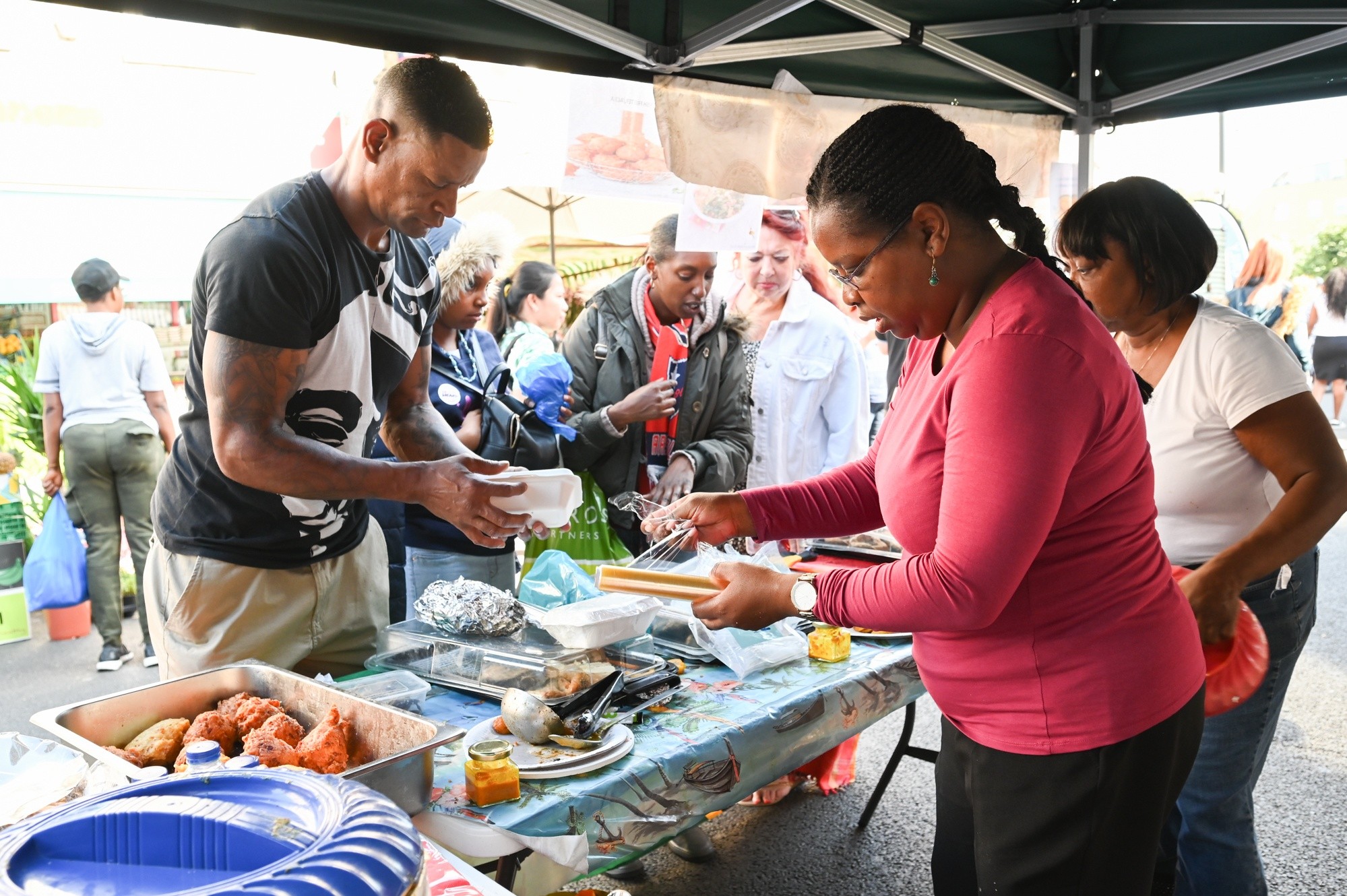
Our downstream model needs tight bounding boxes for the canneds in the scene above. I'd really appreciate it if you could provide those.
[465,740,522,808]
[807,622,851,663]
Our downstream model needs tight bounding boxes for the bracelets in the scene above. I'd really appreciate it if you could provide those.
[46,463,61,467]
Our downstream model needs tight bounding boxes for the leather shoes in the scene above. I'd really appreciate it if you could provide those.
[667,827,713,861]
[603,856,645,878]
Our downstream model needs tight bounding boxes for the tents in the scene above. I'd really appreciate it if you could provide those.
[33,1,1344,207]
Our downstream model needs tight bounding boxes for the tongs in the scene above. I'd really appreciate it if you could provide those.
[548,670,625,740]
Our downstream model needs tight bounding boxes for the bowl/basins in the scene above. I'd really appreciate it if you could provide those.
[469,469,583,530]
[539,592,664,650]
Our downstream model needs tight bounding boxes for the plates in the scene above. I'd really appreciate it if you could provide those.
[841,625,912,639]
[461,713,635,780]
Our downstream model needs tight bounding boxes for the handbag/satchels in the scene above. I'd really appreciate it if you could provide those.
[517,466,635,602]
[22,490,90,614]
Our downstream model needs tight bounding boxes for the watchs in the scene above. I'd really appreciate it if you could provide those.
[789,572,818,620]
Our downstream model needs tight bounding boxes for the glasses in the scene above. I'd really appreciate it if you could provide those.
[771,210,802,227]
[829,212,914,290]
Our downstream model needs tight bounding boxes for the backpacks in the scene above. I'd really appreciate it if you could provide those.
[430,362,560,472]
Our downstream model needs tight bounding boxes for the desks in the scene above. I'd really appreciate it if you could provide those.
[315,551,937,896]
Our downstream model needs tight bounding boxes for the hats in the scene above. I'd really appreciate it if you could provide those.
[71,258,130,292]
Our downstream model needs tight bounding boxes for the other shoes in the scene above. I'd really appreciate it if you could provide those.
[143,643,157,667]
[96,642,134,670]
[1329,419,1347,429]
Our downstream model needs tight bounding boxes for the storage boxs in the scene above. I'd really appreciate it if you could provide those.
[0,586,33,645]
[331,669,432,714]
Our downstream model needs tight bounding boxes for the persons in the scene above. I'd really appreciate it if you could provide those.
[0,451,21,503]
[36,55,1346,896]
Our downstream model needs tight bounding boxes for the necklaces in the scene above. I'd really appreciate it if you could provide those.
[1123,306,1183,374]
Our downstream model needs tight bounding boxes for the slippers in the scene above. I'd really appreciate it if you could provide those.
[738,772,808,806]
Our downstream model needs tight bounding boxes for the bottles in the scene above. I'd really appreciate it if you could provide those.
[465,738,522,809]
[130,739,259,784]
[806,621,851,664]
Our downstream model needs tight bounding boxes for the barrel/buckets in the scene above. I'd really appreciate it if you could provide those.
[0,766,432,896]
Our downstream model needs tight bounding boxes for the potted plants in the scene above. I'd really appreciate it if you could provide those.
[0,329,92,642]
